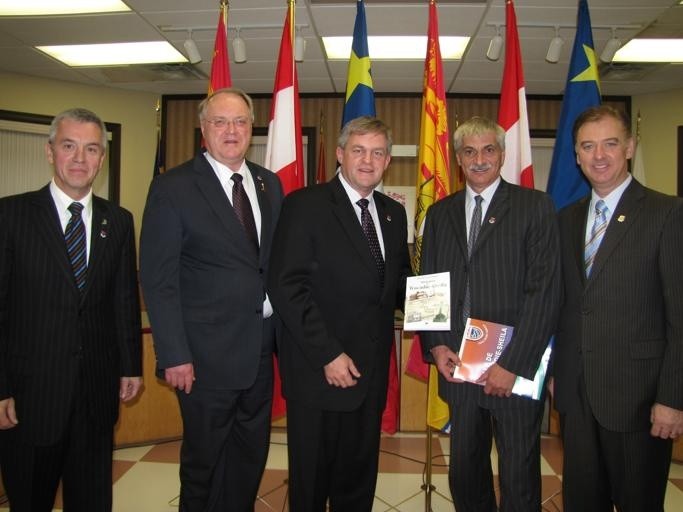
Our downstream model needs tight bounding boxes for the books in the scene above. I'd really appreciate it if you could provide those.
[453,317,557,402]
[403,270,451,332]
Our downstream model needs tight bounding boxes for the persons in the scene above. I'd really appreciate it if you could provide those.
[269,116,415,512]
[139,89,284,512]
[417,116,565,512]
[0,108,145,512]
[547,105,683,512]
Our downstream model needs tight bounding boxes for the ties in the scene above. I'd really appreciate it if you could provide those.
[65,203,88,296]
[463,197,483,326]
[584,200,607,277]
[357,199,383,290]
[231,174,258,252]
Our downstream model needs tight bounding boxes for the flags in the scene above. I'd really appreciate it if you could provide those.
[632,141,646,187]
[153,127,165,176]
[262,2,304,421]
[342,0,401,435]
[547,0,604,215]
[454,110,466,193]
[208,3,233,96]
[406,2,451,435]
[497,1,535,189]
[315,141,326,184]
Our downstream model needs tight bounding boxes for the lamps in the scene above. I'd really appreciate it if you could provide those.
[486,21,641,64]
[159,23,310,65]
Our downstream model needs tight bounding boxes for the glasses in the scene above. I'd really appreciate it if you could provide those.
[205,118,249,127]
